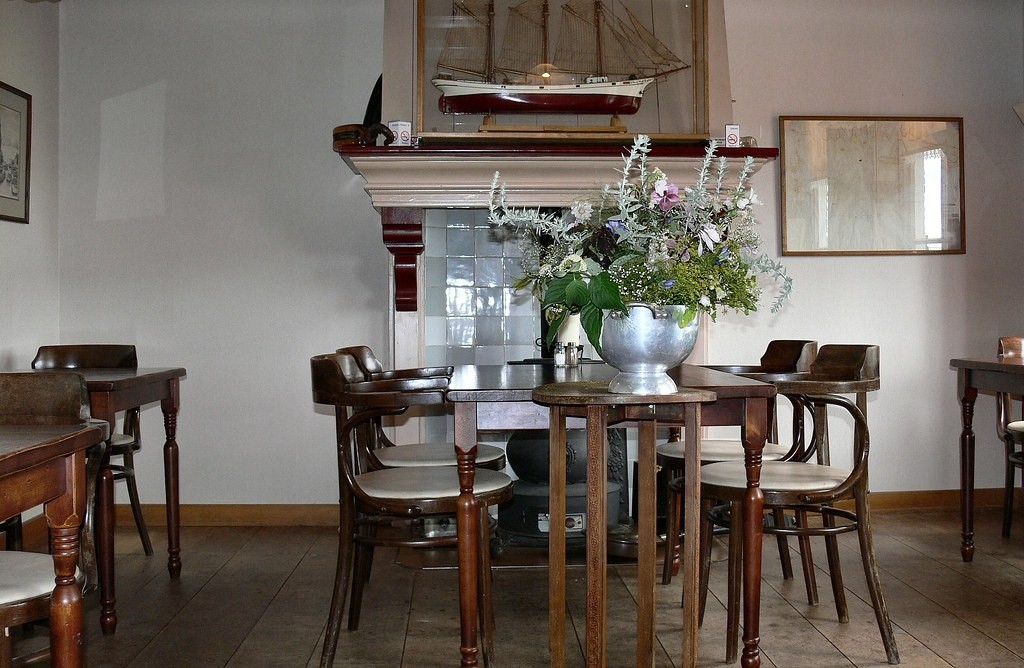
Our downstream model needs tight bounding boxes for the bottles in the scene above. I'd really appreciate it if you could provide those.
[565,342,578,367]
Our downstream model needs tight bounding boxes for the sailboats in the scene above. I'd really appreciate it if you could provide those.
[430,1,691,116]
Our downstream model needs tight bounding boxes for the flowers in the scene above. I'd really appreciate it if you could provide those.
[484,131,796,355]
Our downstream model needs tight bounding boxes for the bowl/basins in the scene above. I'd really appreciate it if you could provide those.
[579,528,664,560]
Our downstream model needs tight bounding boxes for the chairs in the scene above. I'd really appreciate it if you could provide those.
[31,344,158,556]
[0,372,110,632]
[997,338,1024,539]
[309,338,902,668]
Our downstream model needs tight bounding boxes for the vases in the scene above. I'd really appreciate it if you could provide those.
[597,294,701,397]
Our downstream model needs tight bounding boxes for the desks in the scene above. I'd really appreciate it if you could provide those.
[0,423,103,668]
[0,367,187,636]
[949,358,1024,562]
[445,363,762,668]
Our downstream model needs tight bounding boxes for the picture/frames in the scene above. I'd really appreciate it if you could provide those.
[0,80,33,224]
[777,113,967,255]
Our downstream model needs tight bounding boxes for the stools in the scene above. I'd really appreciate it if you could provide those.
[0,551,86,668]
[531,378,717,668]
